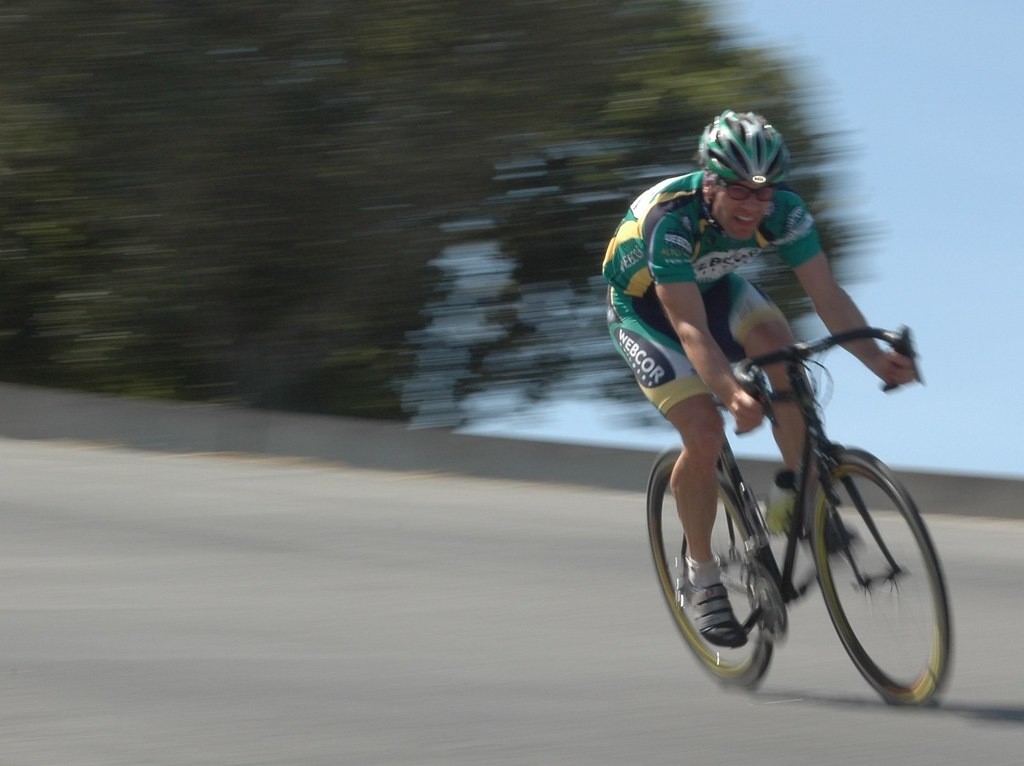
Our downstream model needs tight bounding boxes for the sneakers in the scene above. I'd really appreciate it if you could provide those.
[767,488,851,554]
[682,566,750,664]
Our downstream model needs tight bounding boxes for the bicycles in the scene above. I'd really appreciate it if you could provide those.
[643,324,954,710]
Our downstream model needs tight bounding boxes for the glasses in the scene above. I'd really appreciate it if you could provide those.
[714,175,777,203]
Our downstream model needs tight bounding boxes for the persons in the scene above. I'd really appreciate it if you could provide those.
[603,110,914,648]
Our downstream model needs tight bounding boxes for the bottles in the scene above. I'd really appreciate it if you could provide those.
[767,468,795,532]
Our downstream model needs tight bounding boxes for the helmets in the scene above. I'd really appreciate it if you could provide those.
[698,107,790,185]
